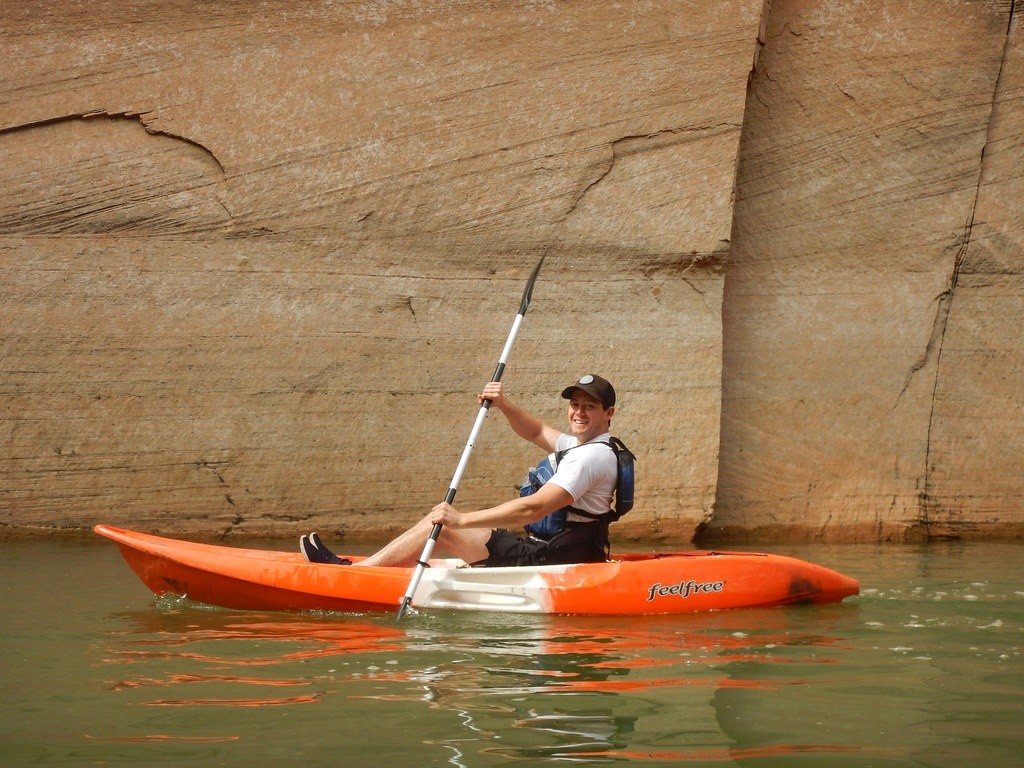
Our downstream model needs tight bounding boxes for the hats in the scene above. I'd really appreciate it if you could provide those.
[562,374,616,405]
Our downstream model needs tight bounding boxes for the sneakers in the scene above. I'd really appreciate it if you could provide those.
[310,532,352,564]
[300,535,341,564]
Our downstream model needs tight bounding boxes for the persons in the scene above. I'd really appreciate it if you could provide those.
[299,375,638,567]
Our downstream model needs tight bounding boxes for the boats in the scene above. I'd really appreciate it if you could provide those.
[93,524,861,614]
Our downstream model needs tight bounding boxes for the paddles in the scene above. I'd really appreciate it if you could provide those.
[391,241,554,624]
